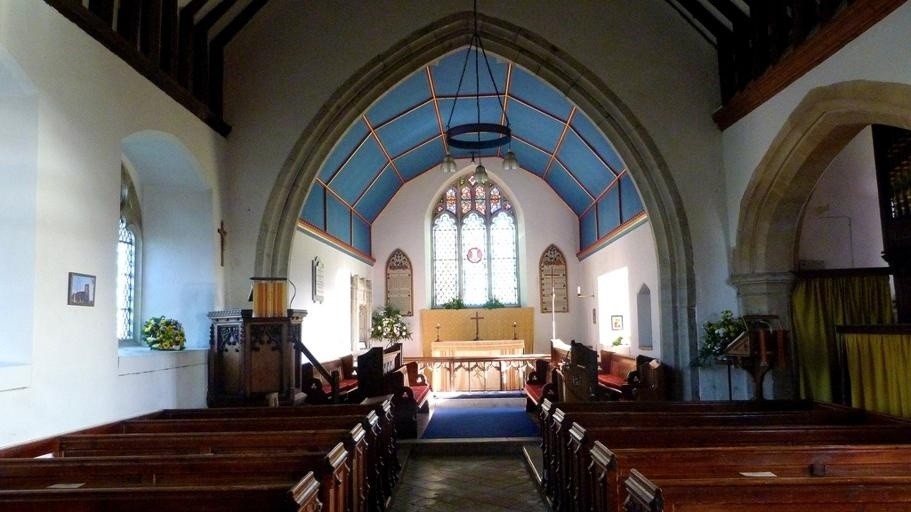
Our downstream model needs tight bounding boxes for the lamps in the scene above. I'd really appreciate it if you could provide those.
[442,1,520,185]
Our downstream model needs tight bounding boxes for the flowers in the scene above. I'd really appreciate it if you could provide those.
[370,302,414,344]
[687,309,744,371]
[144,315,186,350]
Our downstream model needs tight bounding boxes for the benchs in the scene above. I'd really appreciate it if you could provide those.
[303,343,435,438]
[1,396,407,512]
[524,337,673,413]
[535,395,911,511]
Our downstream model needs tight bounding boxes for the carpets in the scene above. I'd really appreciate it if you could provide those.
[422,405,541,438]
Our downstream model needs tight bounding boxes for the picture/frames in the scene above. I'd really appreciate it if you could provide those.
[68,272,97,306]
